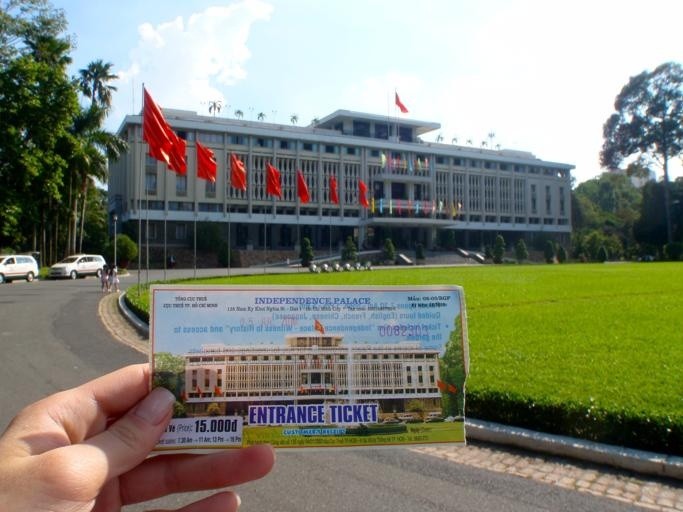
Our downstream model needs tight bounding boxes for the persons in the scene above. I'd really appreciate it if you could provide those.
[106,264,112,282]
[108,264,120,293]
[168,252,176,270]
[0,361,276,512]
[101,265,109,292]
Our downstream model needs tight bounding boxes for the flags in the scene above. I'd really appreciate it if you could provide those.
[447,384,456,393]
[265,163,281,197]
[230,152,245,192]
[369,196,462,220]
[196,139,215,183]
[329,175,338,204]
[215,384,221,397]
[166,132,186,177]
[437,379,446,390]
[357,179,368,209]
[149,116,169,162]
[314,318,324,335]
[378,150,435,174]
[181,389,188,401]
[394,93,410,115]
[140,83,169,143]
[295,170,308,203]
[198,386,202,395]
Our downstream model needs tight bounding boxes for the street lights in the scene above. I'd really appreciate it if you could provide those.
[112,213,118,275]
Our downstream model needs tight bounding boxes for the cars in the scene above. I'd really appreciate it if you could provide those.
[377,410,465,427]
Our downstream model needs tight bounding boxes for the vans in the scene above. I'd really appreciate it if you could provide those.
[0,255,40,284]
[49,253,108,279]
